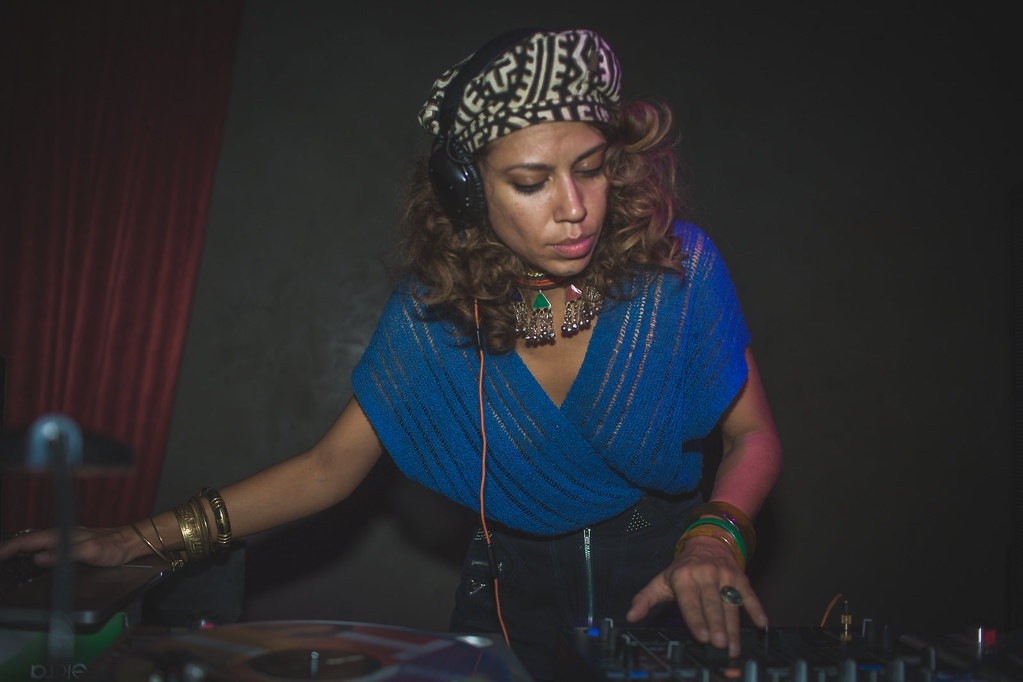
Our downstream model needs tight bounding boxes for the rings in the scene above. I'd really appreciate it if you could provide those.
[719,584,744,609]
[9,527,33,537]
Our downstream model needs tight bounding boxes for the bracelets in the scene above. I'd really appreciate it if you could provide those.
[130,487,231,573]
[672,499,758,571]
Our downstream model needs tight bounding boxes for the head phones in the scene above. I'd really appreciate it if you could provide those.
[429,24,550,231]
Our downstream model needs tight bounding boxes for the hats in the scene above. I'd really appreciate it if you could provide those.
[415,26,625,154]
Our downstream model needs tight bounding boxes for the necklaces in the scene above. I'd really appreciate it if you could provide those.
[509,270,605,343]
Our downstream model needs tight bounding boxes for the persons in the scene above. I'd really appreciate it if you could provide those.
[0,28,779,682]
